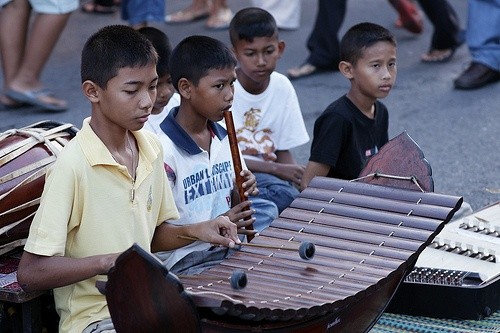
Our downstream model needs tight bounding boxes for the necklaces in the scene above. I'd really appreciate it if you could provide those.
[110,134,135,182]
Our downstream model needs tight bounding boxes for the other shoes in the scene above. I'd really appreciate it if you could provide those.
[454,62,500,89]
[82,2,115,14]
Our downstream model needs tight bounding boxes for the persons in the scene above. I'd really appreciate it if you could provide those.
[121,0,165,30]
[302,22,397,188]
[16,24,241,333]
[388,0,423,33]
[135,27,181,132]
[156,35,258,275]
[164,0,234,28]
[222,8,310,214]
[287,0,466,79]
[0,0,80,111]
[455,0,500,88]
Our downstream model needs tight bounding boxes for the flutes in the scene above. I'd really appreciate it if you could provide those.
[221,109,258,244]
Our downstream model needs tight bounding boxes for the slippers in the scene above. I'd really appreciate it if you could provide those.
[420,27,466,62]
[165,9,209,23]
[5,85,68,110]
[206,9,234,28]
[286,58,327,79]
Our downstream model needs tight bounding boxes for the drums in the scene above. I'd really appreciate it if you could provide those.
[0,118,82,260]
